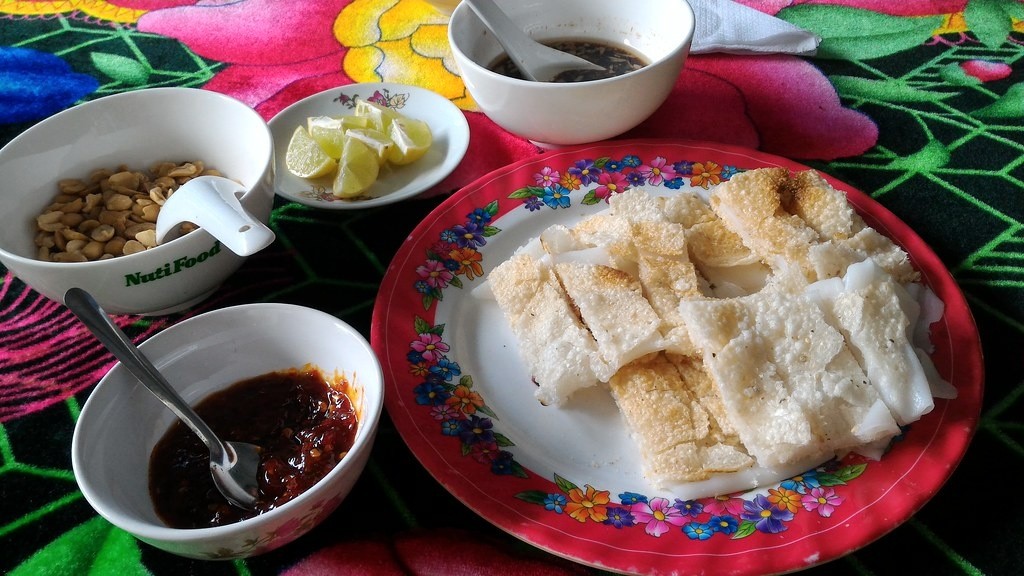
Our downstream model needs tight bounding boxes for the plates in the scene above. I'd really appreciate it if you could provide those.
[265,81,471,208]
[372,140,985,576]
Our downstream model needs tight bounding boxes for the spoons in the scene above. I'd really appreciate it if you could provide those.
[463,0,605,82]
[64,287,260,511]
[155,175,275,257]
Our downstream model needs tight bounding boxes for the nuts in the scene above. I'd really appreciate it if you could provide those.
[26,155,226,264]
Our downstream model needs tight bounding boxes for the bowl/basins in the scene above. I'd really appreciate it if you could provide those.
[0,89,275,315]
[448,1,695,150]
[70,302,385,562]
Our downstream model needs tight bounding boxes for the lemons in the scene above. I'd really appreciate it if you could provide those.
[280,94,433,201]
